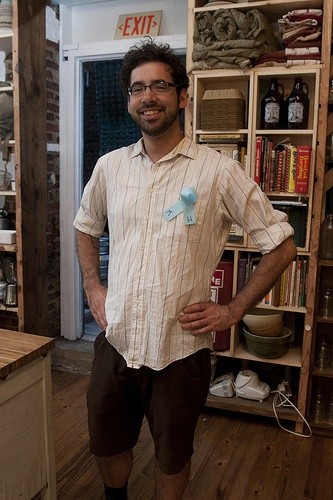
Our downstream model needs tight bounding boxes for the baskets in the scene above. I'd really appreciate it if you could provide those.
[200,99,246,131]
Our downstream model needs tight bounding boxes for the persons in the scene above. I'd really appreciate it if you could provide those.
[73,34,297,500]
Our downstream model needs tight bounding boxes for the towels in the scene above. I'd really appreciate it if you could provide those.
[191,8,276,74]
[277,9,323,68]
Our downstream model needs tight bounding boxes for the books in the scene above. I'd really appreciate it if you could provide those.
[238,255,306,308]
[255,136,312,193]
[199,135,247,171]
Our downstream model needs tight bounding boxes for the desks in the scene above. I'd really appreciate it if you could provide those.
[0,329,57,500]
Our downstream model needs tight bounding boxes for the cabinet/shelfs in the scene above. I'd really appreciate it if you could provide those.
[169,0,333,434]
[308,258,333,433]
[0,0,49,336]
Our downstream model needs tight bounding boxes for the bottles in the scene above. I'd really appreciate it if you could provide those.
[319,210,333,260]
[260,78,285,130]
[285,77,310,130]
[311,389,326,423]
[320,289,331,320]
[316,341,328,370]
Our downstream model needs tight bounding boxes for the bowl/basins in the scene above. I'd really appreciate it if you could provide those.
[243,326,291,359]
[242,310,283,337]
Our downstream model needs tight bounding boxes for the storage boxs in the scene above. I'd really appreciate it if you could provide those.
[1,230,16,245]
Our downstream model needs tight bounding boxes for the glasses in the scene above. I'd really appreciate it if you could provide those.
[128,82,177,96]
